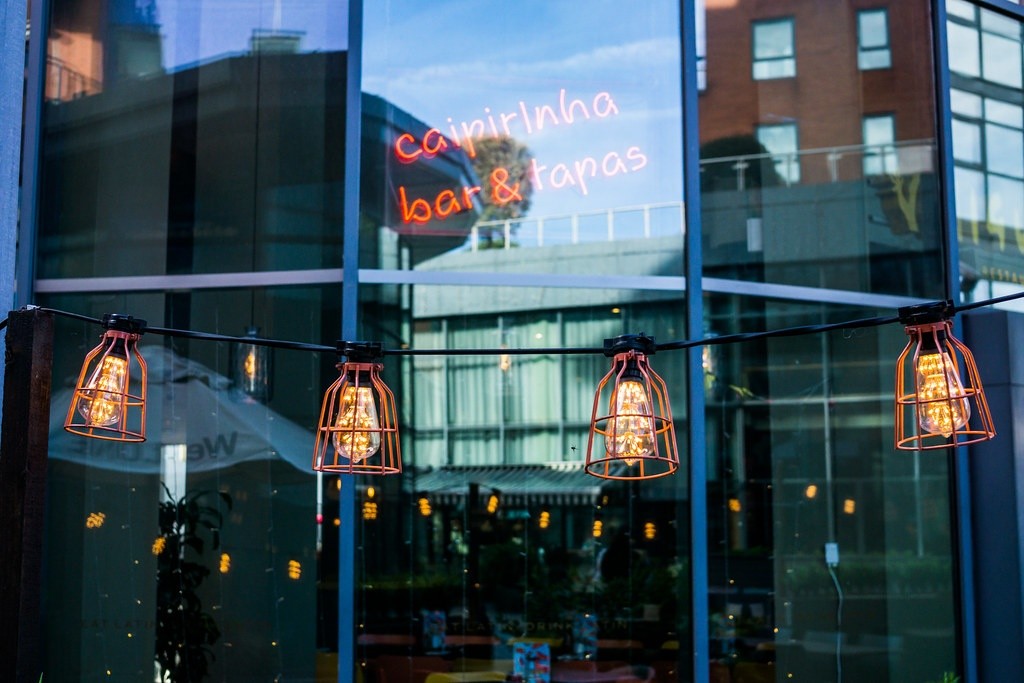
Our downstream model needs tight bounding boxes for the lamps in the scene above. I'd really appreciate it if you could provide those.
[894,298,996,452]
[63,313,148,442]
[228,0,274,408]
[312,340,402,476]
[584,332,680,480]
